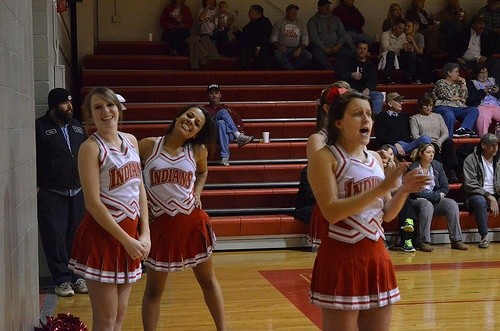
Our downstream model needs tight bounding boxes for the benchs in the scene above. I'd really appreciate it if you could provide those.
[82,40,500,250]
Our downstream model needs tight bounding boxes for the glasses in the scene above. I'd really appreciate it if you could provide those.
[392,8,401,11]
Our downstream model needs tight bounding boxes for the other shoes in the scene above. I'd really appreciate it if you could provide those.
[416,80,421,84]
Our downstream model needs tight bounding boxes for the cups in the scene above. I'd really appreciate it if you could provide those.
[382,92,386,101]
[147,32,153,42]
[357,66,362,73]
[262,132,270,144]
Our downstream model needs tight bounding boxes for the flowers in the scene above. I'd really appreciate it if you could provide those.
[33,313,88,331]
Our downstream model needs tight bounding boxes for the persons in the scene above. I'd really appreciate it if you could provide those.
[335,41,384,117]
[195,0,233,66]
[460,133,500,248]
[446,13,494,75]
[307,91,432,331]
[293,166,316,226]
[201,84,254,166]
[307,0,358,71]
[377,18,436,84]
[410,93,460,183]
[306,81,355,250]
[332,0,379,54]
[268,4,312,70]
[68,86,151,331]
[374,92,434,163]
[376,144,419,252]
[383,0,500,58]
[138,104,226,331]
[465,62,500,138]
[214,1,239,54]
[430,63,480,138]
[159,0,193,55]
[35,88,89,296]
[233,4,272,71]
[405,143,469,252]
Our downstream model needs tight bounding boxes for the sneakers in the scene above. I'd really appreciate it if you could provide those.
[72,278,88,293]
[451,241,468,250]
[465,131,479,137]
[453,128,470,137]
[407,77,416,84]
[384,76,395,84]
[237,131,254,147]
[220,158,229,166]
[400,239,416,252]
[401,218,414,232]
[55,282,74,296]
[421,243,433,252]
[479,238,490,247]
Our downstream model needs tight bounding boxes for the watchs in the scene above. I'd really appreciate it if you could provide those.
[255,47,262,50]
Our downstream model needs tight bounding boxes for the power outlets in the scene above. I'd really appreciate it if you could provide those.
[112,16,120,22]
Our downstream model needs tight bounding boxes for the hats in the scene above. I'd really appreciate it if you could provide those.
[481,133,500,143]
[472,14,486,21]
[48,88,73,111]
[208,83,221,92]
[286,4,299,12]
[318,0,332,5]
